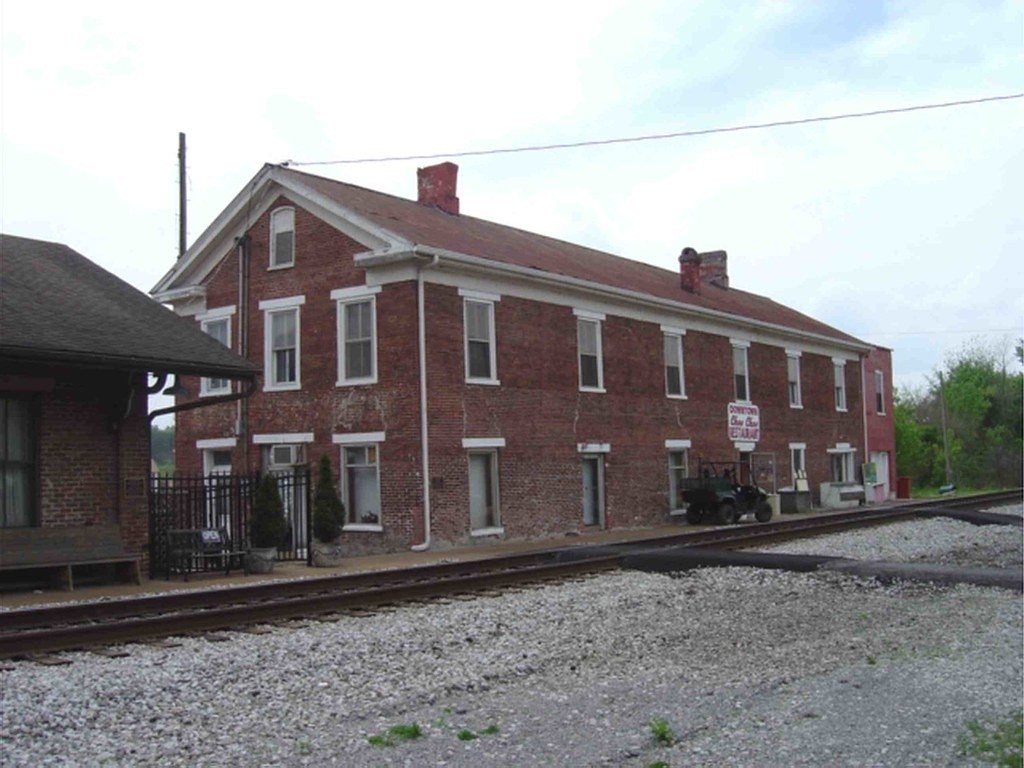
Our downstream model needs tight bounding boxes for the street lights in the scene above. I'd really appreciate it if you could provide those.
[933,382,951,486]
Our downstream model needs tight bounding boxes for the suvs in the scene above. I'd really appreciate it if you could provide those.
[682,462,773,525]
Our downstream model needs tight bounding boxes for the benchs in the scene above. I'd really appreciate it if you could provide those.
[165,528,249,577]
[0,521,143,595]
